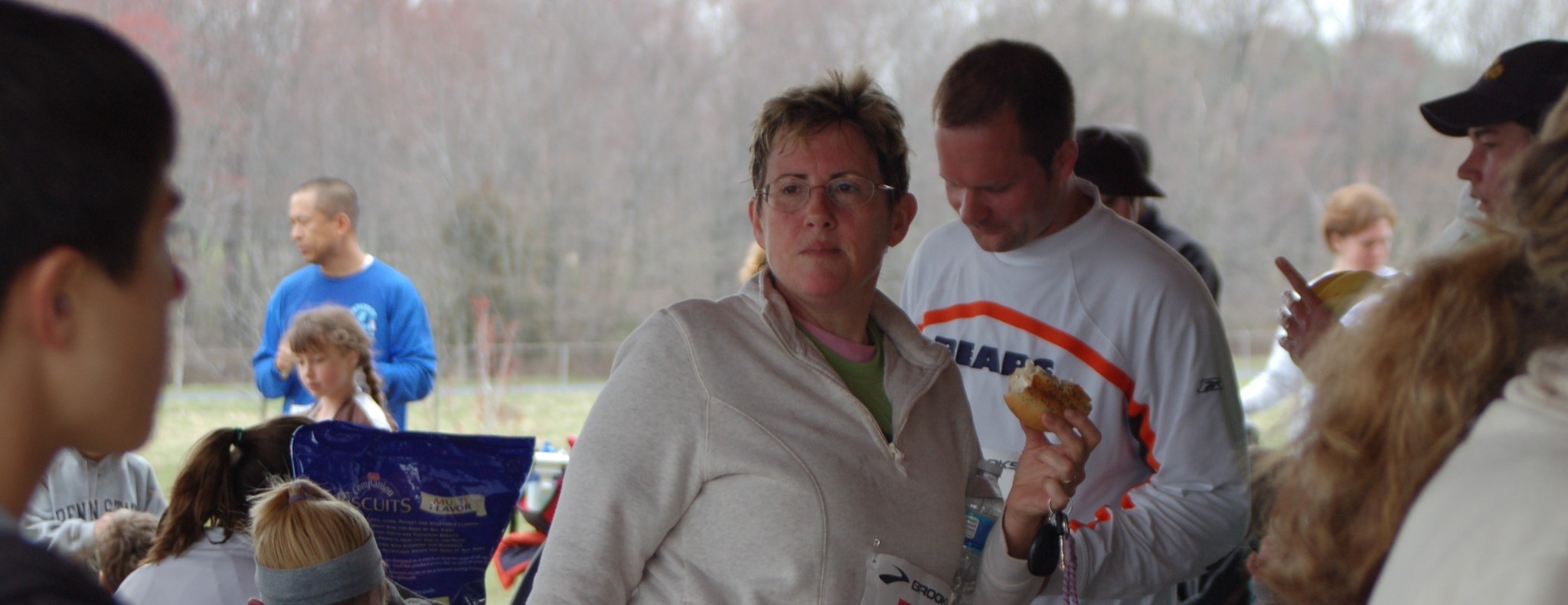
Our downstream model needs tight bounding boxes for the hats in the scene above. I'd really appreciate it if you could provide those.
[1416,39,1568,139]
[1074,126,1159,197]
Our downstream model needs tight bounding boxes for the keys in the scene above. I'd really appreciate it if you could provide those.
[1053,510,1069,572]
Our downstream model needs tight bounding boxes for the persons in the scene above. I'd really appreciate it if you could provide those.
[526,65,1106,605]
[0,0,438,605]
[727,40,1567,605]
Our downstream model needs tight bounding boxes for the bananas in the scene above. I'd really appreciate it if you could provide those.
[1305,269,1406,321]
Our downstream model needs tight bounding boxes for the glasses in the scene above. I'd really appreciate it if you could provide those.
[754,173,898,213]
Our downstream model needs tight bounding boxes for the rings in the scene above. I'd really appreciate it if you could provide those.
[1060,472,1078,486]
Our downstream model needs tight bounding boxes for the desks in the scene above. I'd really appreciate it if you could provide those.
[510,448,571,532]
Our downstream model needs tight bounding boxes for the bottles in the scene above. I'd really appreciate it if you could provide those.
[957,459,1005,602]
[527,473,541,510]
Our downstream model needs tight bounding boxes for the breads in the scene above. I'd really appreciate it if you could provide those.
[1004,359,1092,432]
